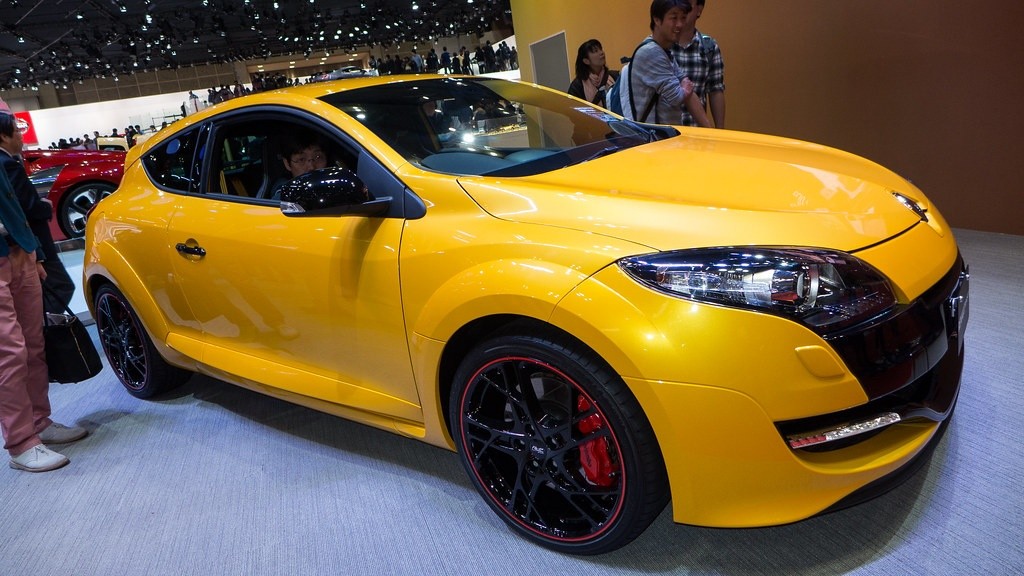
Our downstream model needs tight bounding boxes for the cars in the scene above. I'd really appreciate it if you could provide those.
[83,75,970,556]
[22,150,128,248]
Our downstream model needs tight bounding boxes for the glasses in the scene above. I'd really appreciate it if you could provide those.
[288,154,324,167]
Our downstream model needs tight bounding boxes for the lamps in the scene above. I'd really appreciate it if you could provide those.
[0,0,509,91]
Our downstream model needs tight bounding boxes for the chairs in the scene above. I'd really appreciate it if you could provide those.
[256,134,334,206]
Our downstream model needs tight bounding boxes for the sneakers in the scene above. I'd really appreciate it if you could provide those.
[8,443,70,472]
[38,422,86,443]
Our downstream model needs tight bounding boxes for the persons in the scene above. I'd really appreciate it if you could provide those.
[269,132,327,201]
[47,122,167,150]
[567,39,619,146]
[207,72,313,105]
[646,0,726,129]
[368,40,519,76]
[189,90,198,100]
[0,111,87,472]
[627,0,713,125]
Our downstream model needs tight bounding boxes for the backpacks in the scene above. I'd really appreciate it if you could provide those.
[605,39,668,123]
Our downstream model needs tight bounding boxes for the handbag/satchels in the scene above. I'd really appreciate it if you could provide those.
[39,278,103,384]
